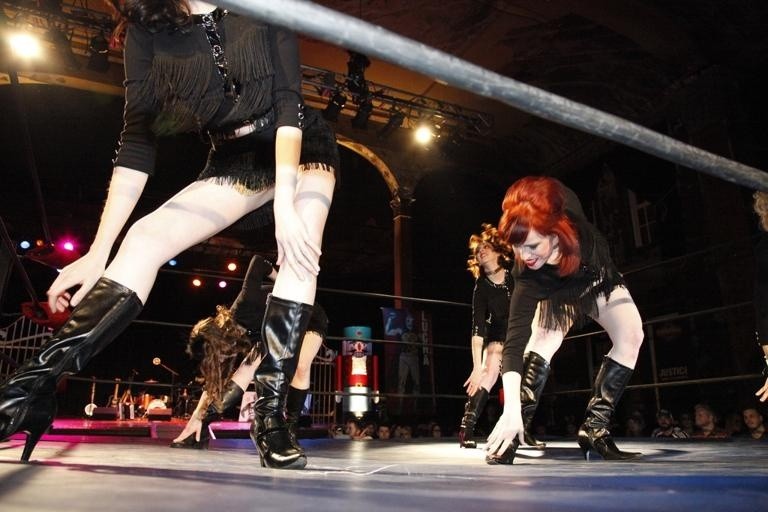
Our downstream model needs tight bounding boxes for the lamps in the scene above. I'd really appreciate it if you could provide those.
[321,89,405,143]
[90,30,109,54]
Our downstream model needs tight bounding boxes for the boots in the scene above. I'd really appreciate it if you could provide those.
[578,355,644,460]
[0,277,143,464]
[170,294,313,469]
[459,351,551,464]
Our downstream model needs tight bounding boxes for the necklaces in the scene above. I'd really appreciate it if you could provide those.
[485,266,502,279]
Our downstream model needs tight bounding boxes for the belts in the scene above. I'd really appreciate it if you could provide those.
[210,112,272,143]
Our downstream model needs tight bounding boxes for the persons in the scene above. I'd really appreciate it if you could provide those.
[344,412,446,439]
[1,0,343,469]
[481,398,767,444]
[483,173,646,465]
[750,191,768,403]
[171,254,331,450]
[458,222,514,448]
